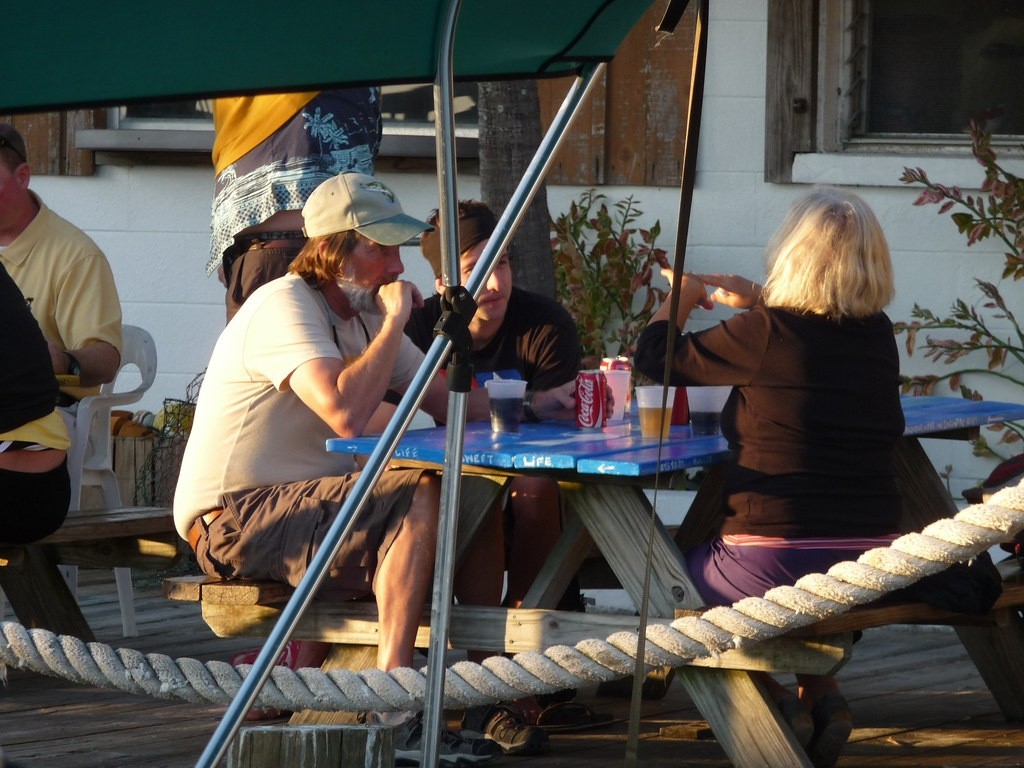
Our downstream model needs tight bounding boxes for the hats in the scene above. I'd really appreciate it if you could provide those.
[301,170,436,246]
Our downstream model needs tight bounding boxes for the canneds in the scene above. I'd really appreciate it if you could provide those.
[599,356,632,413]
[575,369,607,429]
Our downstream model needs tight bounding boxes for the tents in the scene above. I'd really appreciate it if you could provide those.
[0,0,656,120]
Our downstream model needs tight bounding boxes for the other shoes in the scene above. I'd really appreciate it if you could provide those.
[230,639,298,668]
[770,685,814,749]
[245,708,292,721]
[808,695,852,768]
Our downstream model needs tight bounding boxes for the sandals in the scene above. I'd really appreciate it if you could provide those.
[520,690,616,729]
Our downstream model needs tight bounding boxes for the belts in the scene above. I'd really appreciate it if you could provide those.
[186,507,227,555]
[225,238,308,249]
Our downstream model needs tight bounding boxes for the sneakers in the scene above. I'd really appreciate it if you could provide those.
[356,708,503,765]
[461,703,553,756]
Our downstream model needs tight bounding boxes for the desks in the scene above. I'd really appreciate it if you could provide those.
[323,394,1023,673]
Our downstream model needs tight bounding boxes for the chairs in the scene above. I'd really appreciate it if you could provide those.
[48,324,158,636]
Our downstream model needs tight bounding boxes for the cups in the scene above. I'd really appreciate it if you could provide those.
[603,370,632,422]
[634,386,677,439]
[685,386,726,436]
[484,378,527,434]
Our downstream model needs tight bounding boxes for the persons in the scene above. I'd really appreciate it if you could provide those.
[634,191,906,768]
[211,86,382,715]
[172,173,549,768]
[354,202,582,728]
[0,123,123,656]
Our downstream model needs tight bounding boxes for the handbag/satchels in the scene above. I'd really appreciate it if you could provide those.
[891,548,1005,619]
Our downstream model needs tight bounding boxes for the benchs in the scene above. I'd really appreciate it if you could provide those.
[663,555,1023,768]
[0,507,177,630]
[165,522,689,766]
